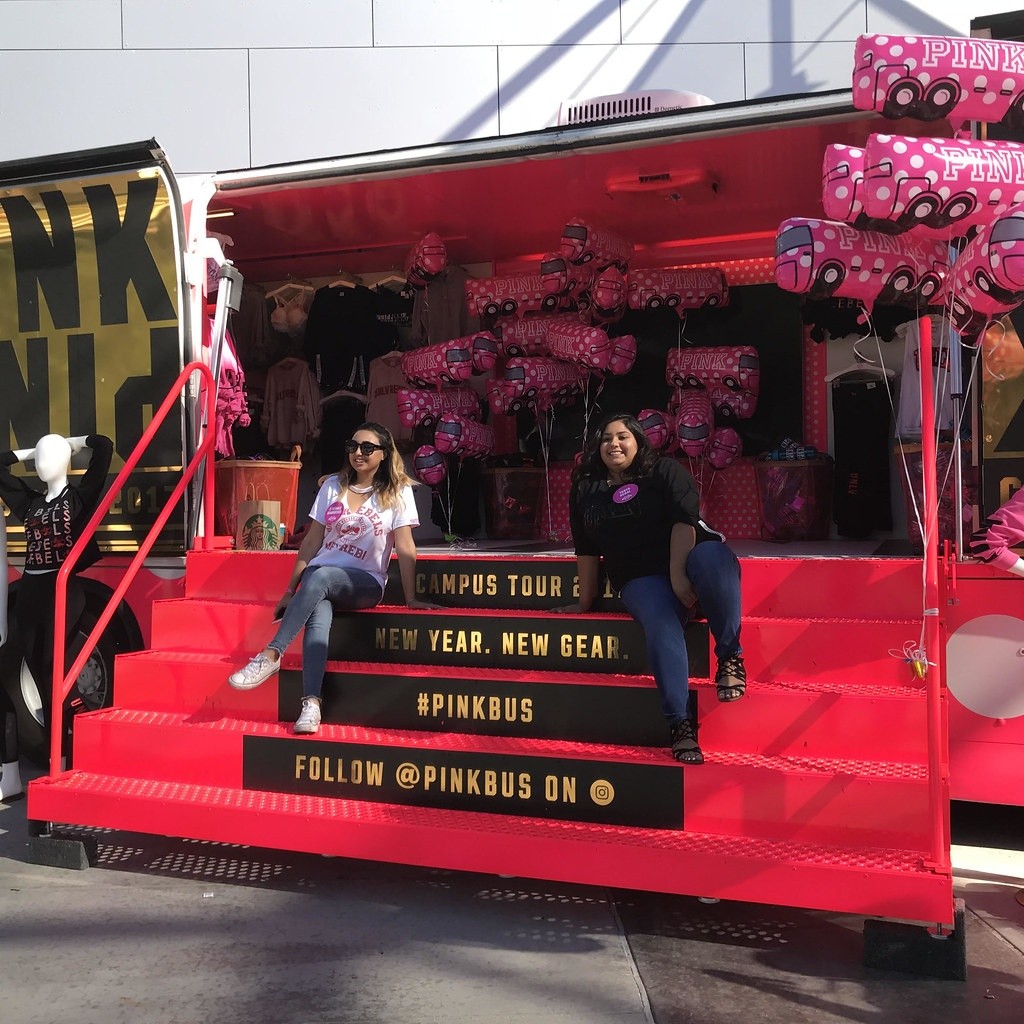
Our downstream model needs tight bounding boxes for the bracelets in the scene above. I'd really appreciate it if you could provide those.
[406,597,415,604]
[285,588,296,595]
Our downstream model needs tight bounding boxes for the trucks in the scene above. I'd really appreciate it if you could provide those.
[1,53,1024,940]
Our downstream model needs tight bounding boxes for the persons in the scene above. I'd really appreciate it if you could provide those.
[969,485,1024,579]
[228,420,446,736]
[1,433,114,805]
[567,411,748,763]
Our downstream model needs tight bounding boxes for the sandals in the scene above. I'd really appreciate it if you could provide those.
[714,656,747,703]
[670,718,704,764]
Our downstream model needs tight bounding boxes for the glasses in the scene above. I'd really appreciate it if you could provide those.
[345,440,385,456]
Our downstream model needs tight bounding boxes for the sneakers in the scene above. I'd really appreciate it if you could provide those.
[228,652,281,691]
[293,700,322,732]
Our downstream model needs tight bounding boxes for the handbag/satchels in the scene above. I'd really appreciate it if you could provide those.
[236,483,281,551]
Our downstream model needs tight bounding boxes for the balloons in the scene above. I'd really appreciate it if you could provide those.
[397,219,762,482]
[774,32,1024,336]
